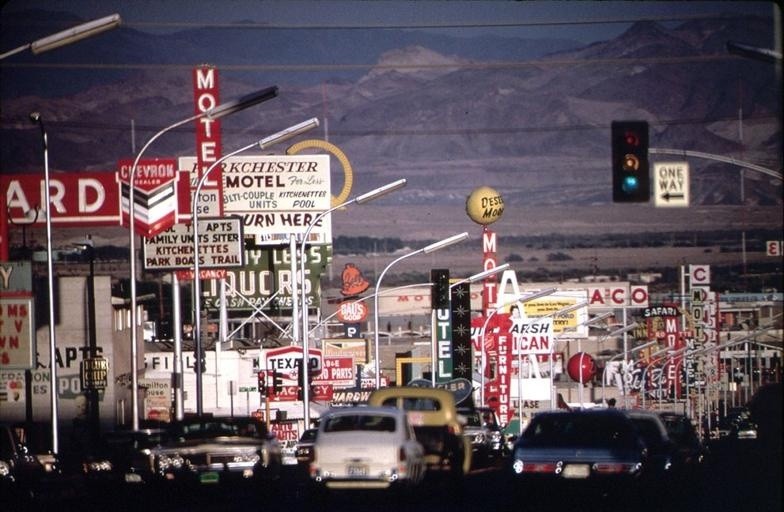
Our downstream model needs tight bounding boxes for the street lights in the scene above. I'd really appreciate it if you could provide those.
[2,14,120,59]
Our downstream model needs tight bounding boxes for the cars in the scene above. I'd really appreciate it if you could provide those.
[512,406,758,501]
[294,386,504,493]
[0,417,281,507]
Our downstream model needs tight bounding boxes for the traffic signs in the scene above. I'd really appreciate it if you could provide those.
[653,163,689,207]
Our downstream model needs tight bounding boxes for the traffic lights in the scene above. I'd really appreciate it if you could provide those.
[611,117,653,202]
